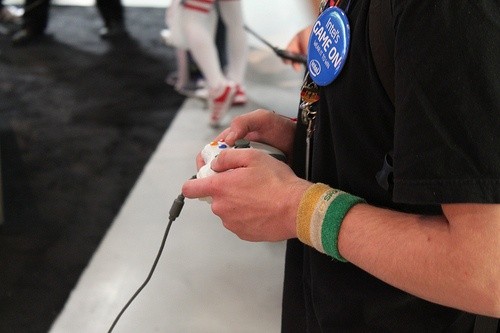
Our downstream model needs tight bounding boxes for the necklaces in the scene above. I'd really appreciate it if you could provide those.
[298,0,339,125]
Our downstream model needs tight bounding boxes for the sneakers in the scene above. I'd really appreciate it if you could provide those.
[229,78,246,104]
[208,83,236,126]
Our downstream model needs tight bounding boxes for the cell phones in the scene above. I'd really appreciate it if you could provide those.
[243,25,308,63]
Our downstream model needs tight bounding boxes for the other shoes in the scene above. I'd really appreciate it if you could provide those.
[97,25,128,39]
[13,23,43,42]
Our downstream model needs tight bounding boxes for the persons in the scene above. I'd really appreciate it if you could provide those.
[9,0,141,69]
[183,0,499,332]
[181,0,247,131]
